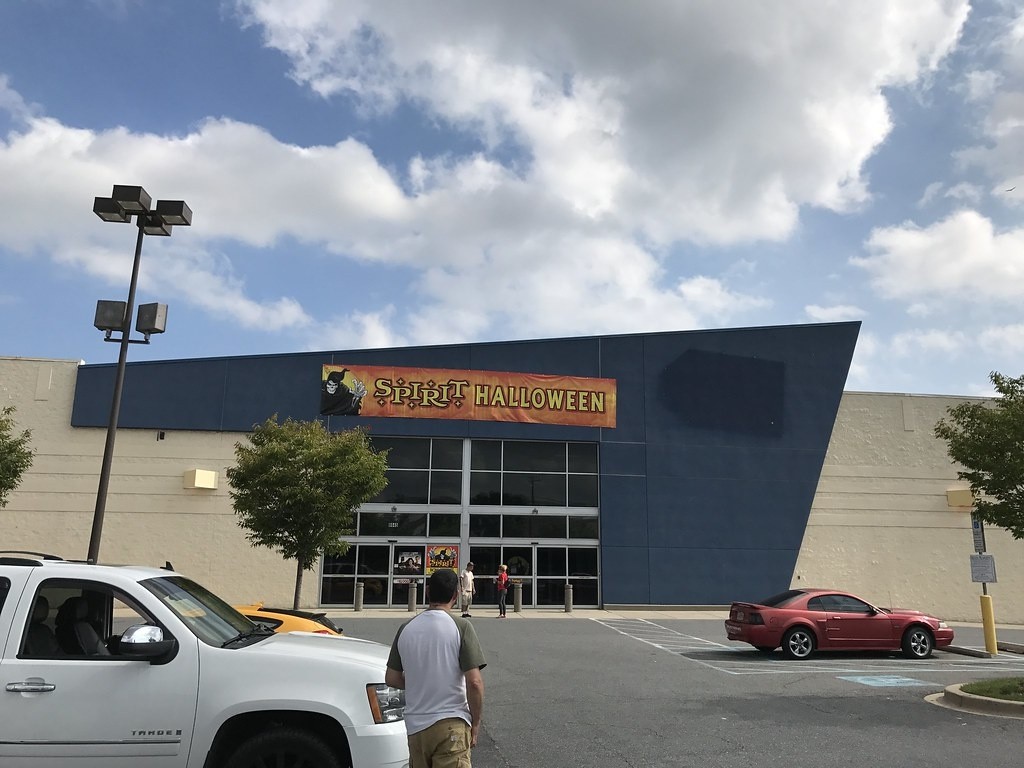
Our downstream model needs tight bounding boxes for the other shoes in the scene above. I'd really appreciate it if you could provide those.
[462,614,471,618]
[496,615,506,619]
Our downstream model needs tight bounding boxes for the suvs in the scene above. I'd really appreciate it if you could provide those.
[0,548,411,768]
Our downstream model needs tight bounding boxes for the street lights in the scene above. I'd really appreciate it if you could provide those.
[85,184,193,563]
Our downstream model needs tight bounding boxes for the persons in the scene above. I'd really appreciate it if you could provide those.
[460,562,476,618]
[493,565,508,618]
[385,569,488,768]
[405,558,418,570]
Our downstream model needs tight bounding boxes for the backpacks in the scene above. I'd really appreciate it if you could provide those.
[504,578,511,588]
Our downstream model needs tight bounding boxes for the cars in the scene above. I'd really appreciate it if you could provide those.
[724,588,955,661]
[144,602,343,637]
